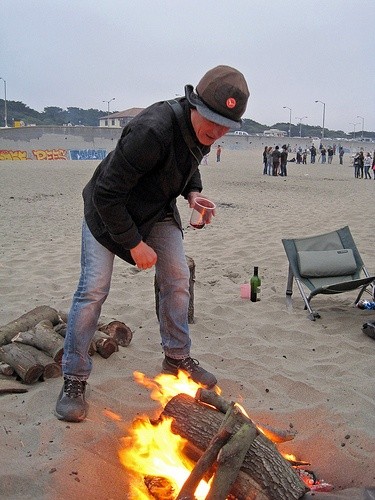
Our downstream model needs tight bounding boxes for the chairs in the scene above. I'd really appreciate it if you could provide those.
[282,226,375,321]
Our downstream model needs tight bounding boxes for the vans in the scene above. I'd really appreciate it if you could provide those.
[233,131,249,136]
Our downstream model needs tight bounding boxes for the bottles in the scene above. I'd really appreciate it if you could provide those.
[250,266,261,302]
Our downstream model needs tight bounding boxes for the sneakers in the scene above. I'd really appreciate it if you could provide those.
[161,356,217,390]
[54,379,88,422]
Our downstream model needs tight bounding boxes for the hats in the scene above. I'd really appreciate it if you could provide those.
[184,65,249,129]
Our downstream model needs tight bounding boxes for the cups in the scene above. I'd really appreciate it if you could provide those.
[189,197,216,229]
[240,284,250,299]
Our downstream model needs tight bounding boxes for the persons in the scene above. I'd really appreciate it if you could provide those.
[216,144,221,162]
[261,142,375,181]
[52,64,251,422]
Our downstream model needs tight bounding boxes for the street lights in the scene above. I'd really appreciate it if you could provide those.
[295,116,308,137]
[349,122,360,140]
[0,77,7,127]
[102,97,115,128]
[357,116,364,138]
[282,106,292,137]
[314,100,325,144]
